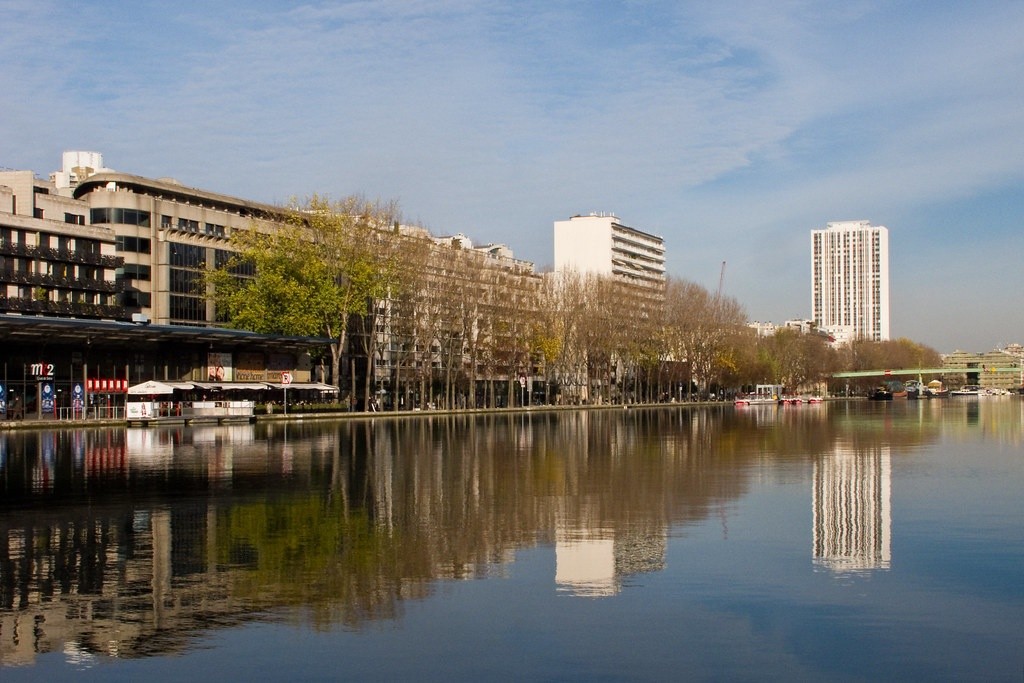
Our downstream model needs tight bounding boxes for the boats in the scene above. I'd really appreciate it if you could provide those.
[951,389,978,395]
[922,389,949,398]
